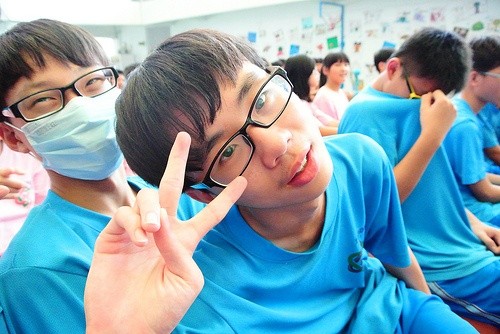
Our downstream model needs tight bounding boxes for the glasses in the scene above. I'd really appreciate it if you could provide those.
[399,64,422,99]
[187,65,294,193]
[0,64,121,124]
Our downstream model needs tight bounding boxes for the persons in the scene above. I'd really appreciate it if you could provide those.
[271,34,500,334]
[0,19,212,334]
[0,133,50,255]
[106,69,126,90]
[82,27,481,334]
